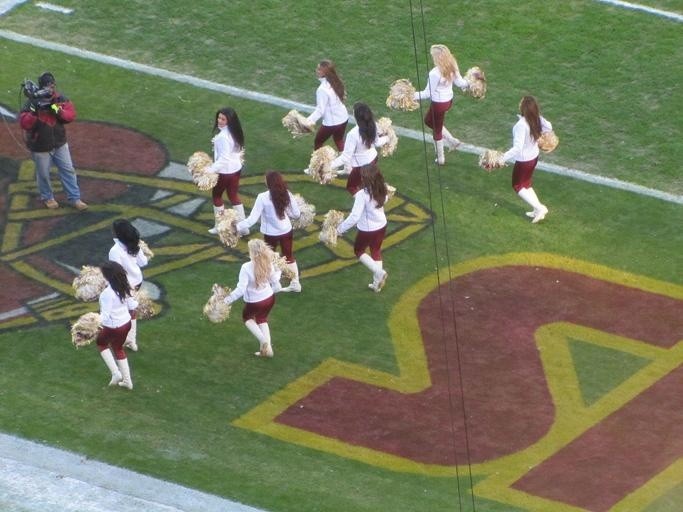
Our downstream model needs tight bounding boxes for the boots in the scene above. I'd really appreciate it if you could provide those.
[518,187,548,224]
[244,255,387,360]
[442,127,459,154]
[434,138,445,166]
[100,320,138,390]
[337,161,353,176]
[208,204,224,235]
[526,188,544,220]
[231,203,249,236]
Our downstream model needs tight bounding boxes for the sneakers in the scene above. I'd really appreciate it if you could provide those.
[73,200,88,210]
[44,198,58,209]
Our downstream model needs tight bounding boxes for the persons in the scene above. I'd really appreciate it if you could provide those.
[503,95,553,224]
[96,258,135,391]
[334,100,390,206]
[225,237,282,358]
[20,72,89,212]
[108,218,149,352]
[235,170,303,293]
[336,164,389,293]
[305,60,349,152]
[206,106,251,237]
[412,44,468,166]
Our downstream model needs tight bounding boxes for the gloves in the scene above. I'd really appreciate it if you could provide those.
[48,103,58,114]
[29,98,40,113]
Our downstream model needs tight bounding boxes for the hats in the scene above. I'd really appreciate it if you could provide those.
[38,71,55,89]
